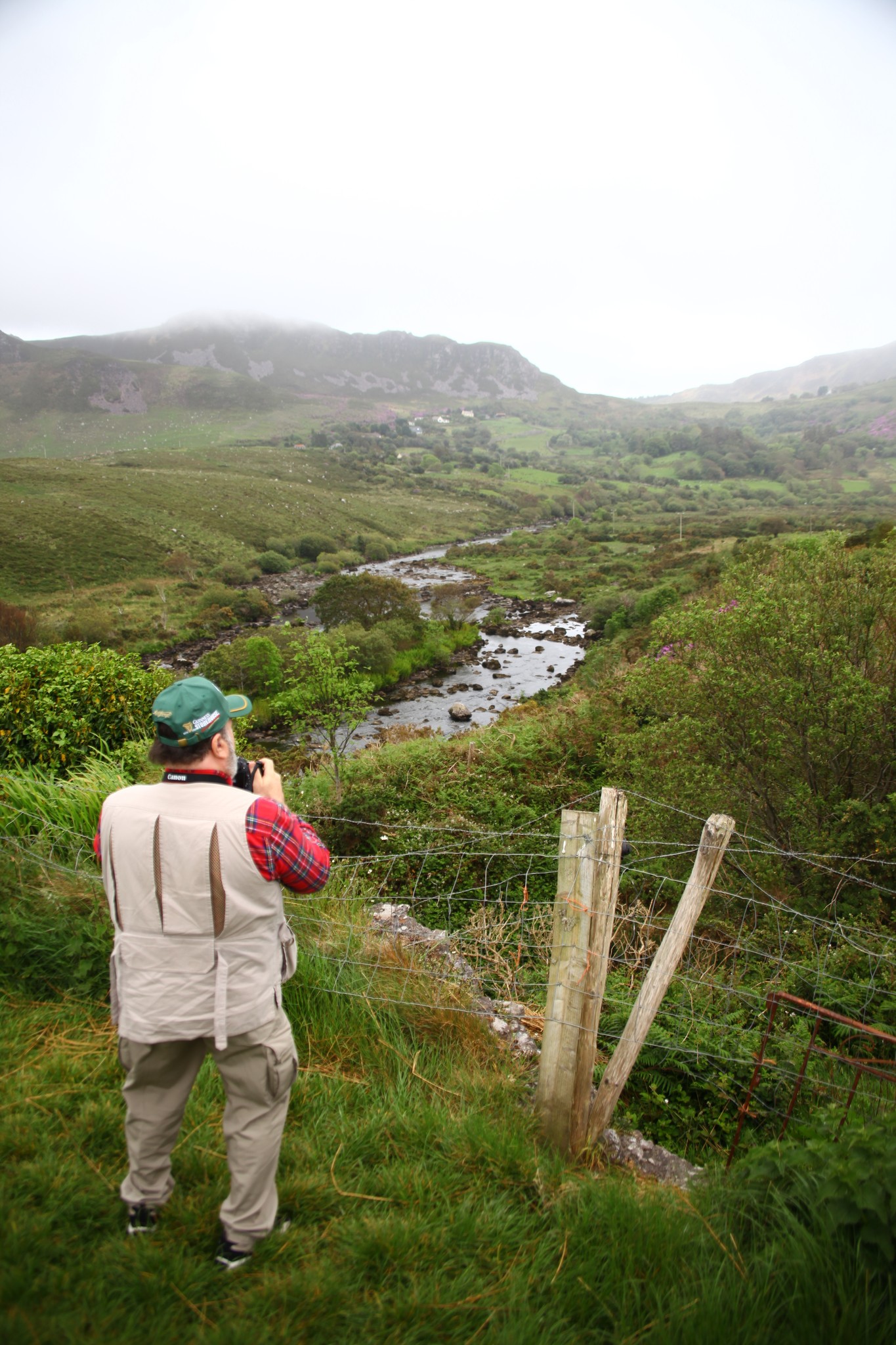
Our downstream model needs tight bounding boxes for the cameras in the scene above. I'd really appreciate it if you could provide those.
[230,756,262,793]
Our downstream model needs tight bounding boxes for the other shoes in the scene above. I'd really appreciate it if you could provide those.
[216,1209,293,1273]
[126,1202,164,1232]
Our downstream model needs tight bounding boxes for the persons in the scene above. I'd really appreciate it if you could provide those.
[94,673,332,1268]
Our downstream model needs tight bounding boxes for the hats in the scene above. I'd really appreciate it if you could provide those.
[152,675,253,748]
